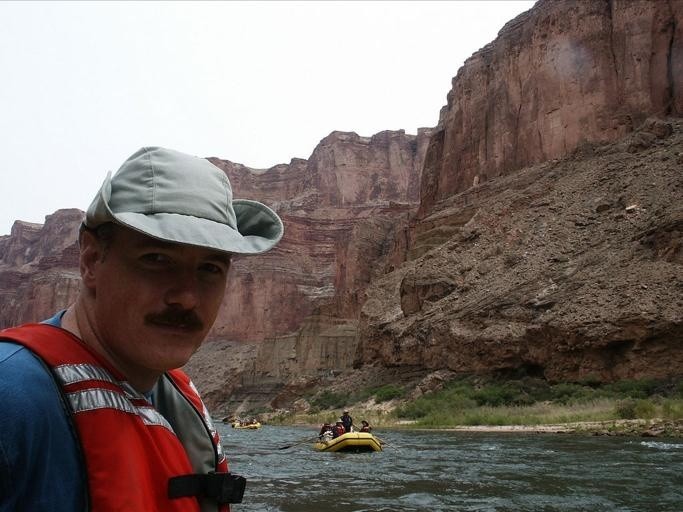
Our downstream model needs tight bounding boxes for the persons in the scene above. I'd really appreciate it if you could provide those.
[0,145,287,512]
[238,417,257,428]
[318,408,371,442]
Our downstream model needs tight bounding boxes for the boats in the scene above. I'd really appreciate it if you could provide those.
[231,422,262,429]
[315,432,382,453]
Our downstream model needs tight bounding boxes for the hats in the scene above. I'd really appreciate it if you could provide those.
[362,420,369,427]
[82,142,286,256]
[341,410,350,415]
[323,422,332,427]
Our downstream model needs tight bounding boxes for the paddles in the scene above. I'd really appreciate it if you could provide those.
[352,424,400,452]
[278,432,331,450]
